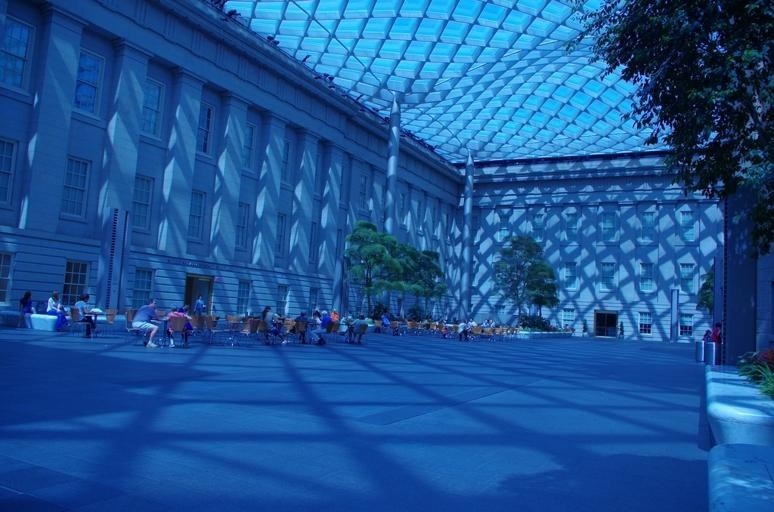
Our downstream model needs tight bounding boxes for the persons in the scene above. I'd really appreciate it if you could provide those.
[20,290,102,335]
[703,330,712,342]
[131,294,207,349]
[261,304,367,349]
[710,322,721,342]
[377,313,496,342]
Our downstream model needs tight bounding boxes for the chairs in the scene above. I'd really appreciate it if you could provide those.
[17,298,369,351]
[374,318,519,344]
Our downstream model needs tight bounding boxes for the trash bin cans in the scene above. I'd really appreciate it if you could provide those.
[704,342,721,365]
[696,342,704,363]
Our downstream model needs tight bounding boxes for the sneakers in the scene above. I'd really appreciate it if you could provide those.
[146,342,158,348]
